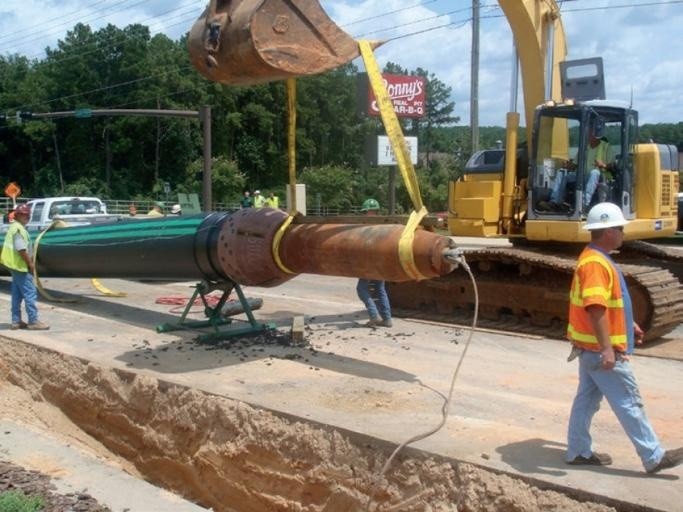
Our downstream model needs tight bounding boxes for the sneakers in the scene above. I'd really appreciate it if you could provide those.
[12,321,49,330]
[569,453,612,466]
[648,447,683,473]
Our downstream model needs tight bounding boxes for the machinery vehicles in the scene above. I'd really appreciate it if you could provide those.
[188,0,683,346]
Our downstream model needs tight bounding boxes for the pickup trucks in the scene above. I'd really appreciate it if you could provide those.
[2,196,128,235]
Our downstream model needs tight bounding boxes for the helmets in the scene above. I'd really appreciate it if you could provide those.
[15,203,29,215]
[359,198,381,212]
[582,202,631,230]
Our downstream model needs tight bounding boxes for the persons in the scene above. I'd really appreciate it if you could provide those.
[129,202,181,218]
[0,204,50,330]
[9,205,16,222]
[567,203,683,474]
[538,135,614,212]
[357,199,392,327]
[239,190,279,209]
